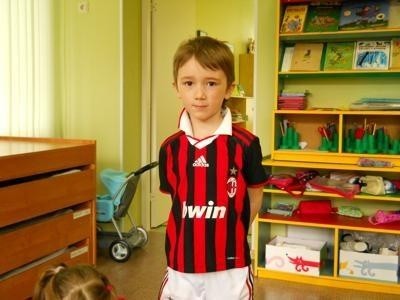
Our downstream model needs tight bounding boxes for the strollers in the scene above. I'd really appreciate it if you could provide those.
[95,161,160,262]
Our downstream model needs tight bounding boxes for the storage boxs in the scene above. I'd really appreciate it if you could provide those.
[265,235,327,276]
[340,238,400,282]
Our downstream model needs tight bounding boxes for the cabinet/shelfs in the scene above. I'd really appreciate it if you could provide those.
[251,158,400,300]
[274,0,400,167]
[1,139,96,300]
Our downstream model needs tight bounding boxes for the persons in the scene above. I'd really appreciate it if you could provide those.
[154,35,274,300]
[30,261,127,299]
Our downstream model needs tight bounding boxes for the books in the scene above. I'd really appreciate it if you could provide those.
[276,0,399,115]
